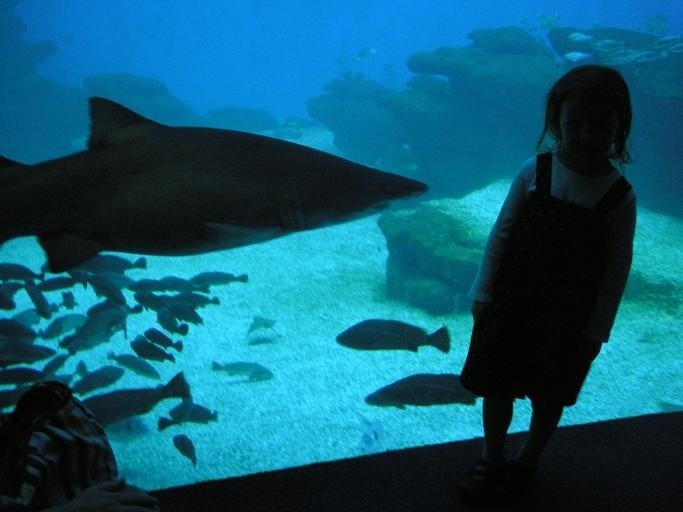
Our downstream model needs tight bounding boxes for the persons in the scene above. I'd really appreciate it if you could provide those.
[460,64,638,507]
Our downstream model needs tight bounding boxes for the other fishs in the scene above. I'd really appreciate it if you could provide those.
[0,251,248,467]
